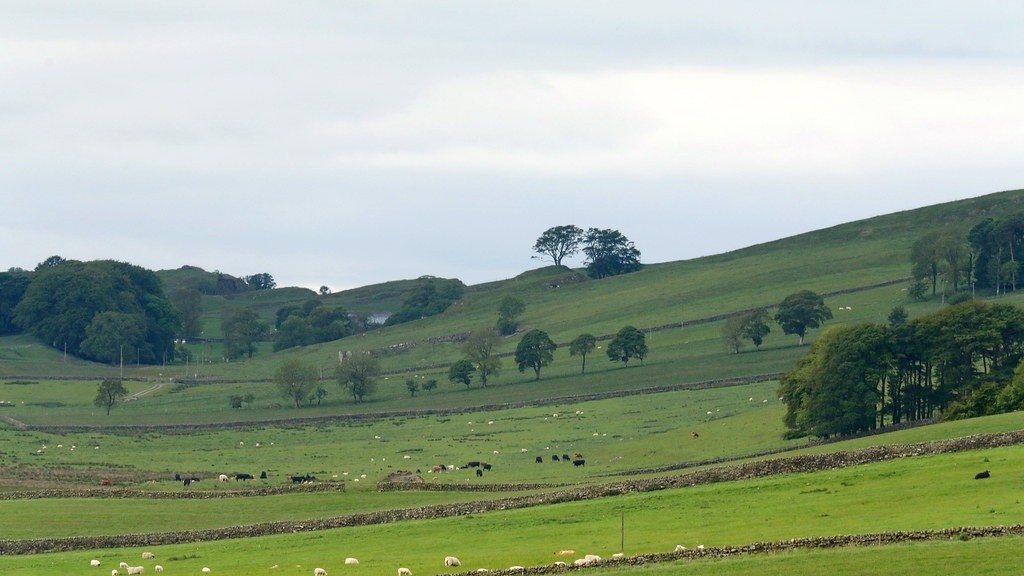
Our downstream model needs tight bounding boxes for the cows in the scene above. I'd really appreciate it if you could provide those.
[174,470,316,484]
[535,452,585,467]
[975,469,992,478]
[417,461,492,477]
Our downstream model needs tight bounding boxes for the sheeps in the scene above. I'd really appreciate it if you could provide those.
[345,558,359,565]
[398,568,413,576]
[445,545,703,572]
[202,567,210,572]
[314,568,328,576]
[88,551,168,575]
